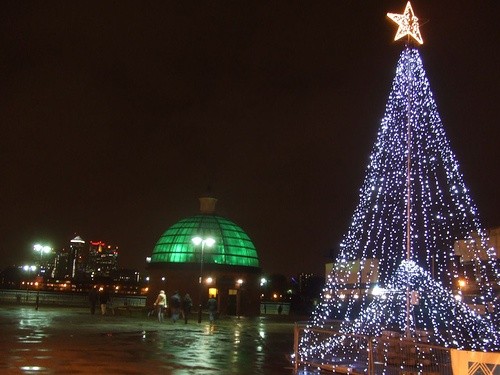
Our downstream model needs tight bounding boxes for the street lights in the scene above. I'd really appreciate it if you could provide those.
[23,265,36,301]
[236,278,243,320]
[34,243,51,311]
[191,236,216,324]
[260,277,267,316]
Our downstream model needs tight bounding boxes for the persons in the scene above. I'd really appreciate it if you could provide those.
[88,288,110,316]
[182,293,193,324]
[171,292,181,323]
[154,290,167,323]
[207,295,217,323]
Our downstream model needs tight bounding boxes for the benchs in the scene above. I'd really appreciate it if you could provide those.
[110,295,147,316]
